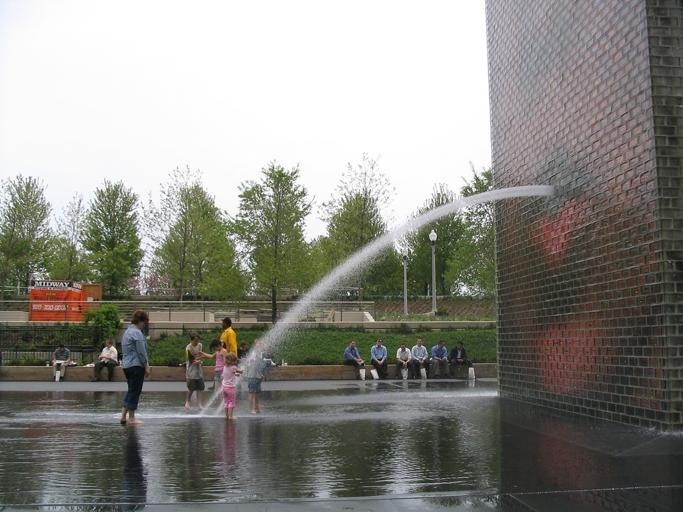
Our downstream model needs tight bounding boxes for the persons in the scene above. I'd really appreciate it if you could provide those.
[395,342,416,379]
[185,317,278,420]
[120,310,151,425]
[370,337,389,380]
[431,340,454,379]
[51,344,71,382]
[448,340,472,379]
[90,338,118,381]
[343,341,369,380]
[411,337,430,378]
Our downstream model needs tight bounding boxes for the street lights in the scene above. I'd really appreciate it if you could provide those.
[401,242,411,314]
[428,227,439,315]
